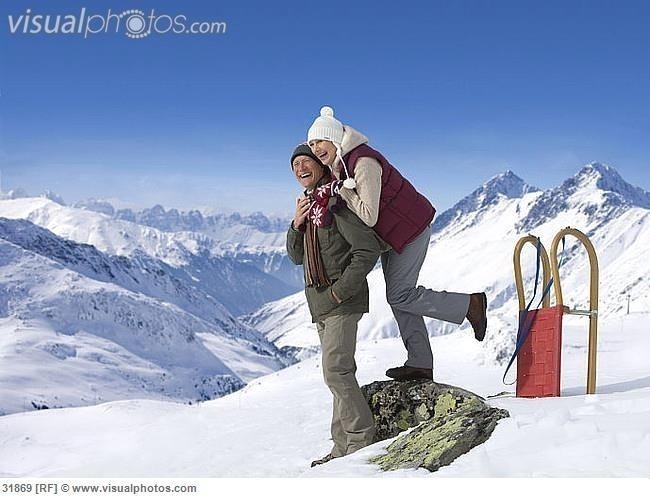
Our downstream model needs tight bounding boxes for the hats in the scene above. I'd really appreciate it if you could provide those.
[306,106,344,146]
[290,141,324,170]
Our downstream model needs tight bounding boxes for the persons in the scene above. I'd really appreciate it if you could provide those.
[305,106,489,380]
[287,141,381,468]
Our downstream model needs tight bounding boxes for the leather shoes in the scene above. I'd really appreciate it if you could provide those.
[385,365,433,381]
[465,292,488,340]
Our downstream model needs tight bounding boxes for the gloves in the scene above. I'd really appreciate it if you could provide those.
[307,201,332,228]
[314,180,344,205]
